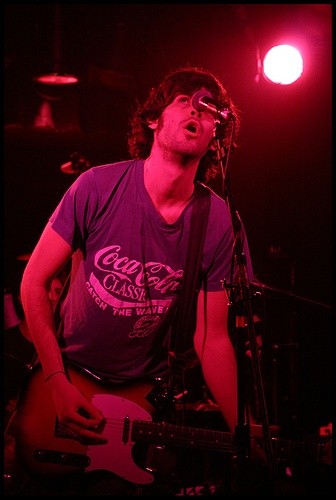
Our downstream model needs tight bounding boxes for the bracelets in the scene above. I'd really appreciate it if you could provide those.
[42,370,67,383]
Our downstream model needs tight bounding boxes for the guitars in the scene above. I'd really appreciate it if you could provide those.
[14,356,335,486]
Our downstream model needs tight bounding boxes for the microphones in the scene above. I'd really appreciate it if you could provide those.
[192,90,233,122]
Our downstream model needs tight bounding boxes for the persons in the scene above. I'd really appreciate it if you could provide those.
[20,61,283,499]
[0,268,64,428]
